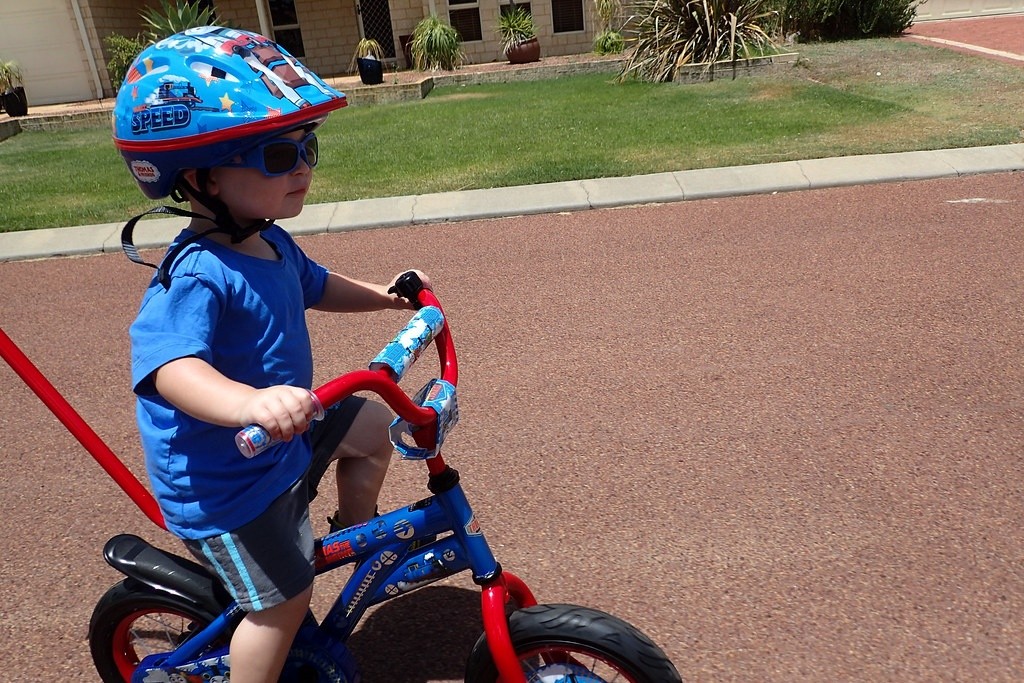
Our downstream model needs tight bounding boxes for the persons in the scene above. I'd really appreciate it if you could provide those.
[112,25,433,683]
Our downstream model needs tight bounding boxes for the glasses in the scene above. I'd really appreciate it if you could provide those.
[215,131,320,177]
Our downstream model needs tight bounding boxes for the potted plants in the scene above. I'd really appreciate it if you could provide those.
[0,56,30,117]
[345,38,389,85]
[490,6,543,65]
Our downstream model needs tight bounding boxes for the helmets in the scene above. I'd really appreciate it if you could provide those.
[110,25,348,199]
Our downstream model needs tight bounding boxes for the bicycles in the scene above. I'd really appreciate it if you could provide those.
[86,272,687,683]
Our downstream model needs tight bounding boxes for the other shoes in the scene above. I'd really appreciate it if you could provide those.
[327,505,437,564]
